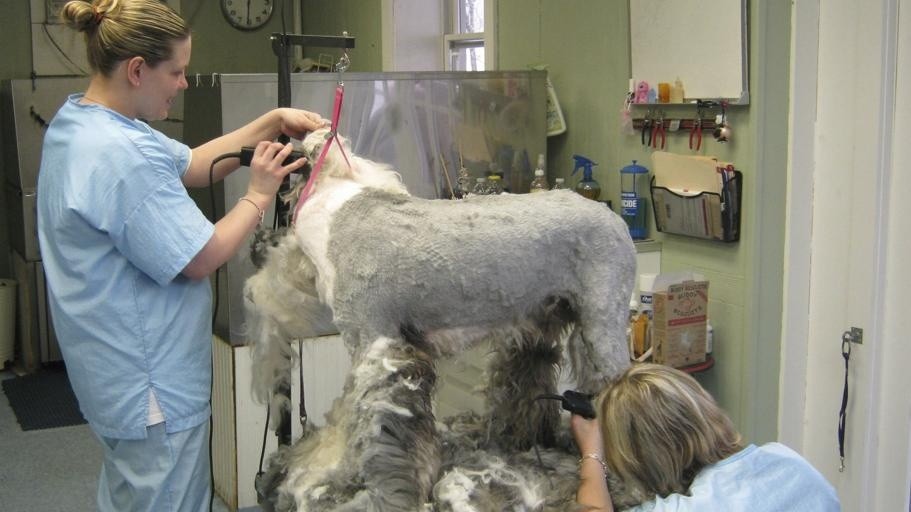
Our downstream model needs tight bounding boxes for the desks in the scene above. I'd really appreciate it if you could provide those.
[677,354,714,382]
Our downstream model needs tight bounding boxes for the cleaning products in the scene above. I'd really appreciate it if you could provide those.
[569,154,602,202]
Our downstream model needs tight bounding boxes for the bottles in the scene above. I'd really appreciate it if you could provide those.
[639,275,658,317]
[618,160,651,240]
[530,168,548,193]
[636,81,649,104]
[672,75,685,104]
[485,175,501,194]
[471,177,486,196]
[648,87,657,104]
[549,178,564,191]
[704,320,713,355]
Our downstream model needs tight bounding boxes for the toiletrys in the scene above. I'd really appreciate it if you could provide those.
[628,77,685,103]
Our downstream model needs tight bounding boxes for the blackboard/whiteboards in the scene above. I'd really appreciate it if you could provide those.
[629,0,749,105]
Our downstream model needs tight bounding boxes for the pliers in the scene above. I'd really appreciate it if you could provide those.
[689,108,702,151]
[652,110,665,150]
[641,109,652,146]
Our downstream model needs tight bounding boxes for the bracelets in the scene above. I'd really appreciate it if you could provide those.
[576,453,607,476]
[239,195,266,224]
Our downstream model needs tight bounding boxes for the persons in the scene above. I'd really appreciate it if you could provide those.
[573,361,841,512]
[34,0,333,512]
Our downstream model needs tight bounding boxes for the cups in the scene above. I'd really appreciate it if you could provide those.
[657,82,670,102]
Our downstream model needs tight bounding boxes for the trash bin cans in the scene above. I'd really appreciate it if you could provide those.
[0,278,18,370]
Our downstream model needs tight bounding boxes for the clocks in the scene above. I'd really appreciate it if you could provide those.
[220,2,275,32]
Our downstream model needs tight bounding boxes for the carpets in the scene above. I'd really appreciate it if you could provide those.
[4,365,86,433]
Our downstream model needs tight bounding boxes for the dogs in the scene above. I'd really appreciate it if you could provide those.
[291,118,638,512]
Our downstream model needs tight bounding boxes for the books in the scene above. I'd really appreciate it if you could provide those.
[650,152,738,244]
